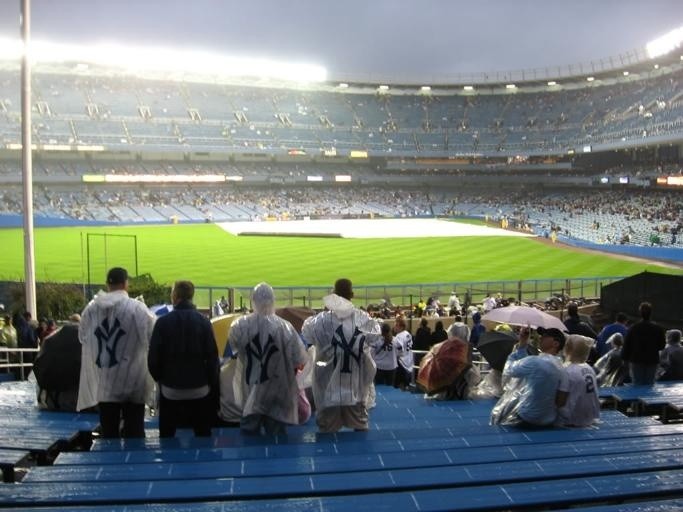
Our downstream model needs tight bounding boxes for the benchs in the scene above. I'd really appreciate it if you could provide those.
[0,67,681,263]
[1,347,683,511]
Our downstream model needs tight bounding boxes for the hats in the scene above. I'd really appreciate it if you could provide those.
[536,326,565,343]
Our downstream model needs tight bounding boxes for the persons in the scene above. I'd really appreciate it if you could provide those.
[0,268,680,441]
[3,59,679,251]
